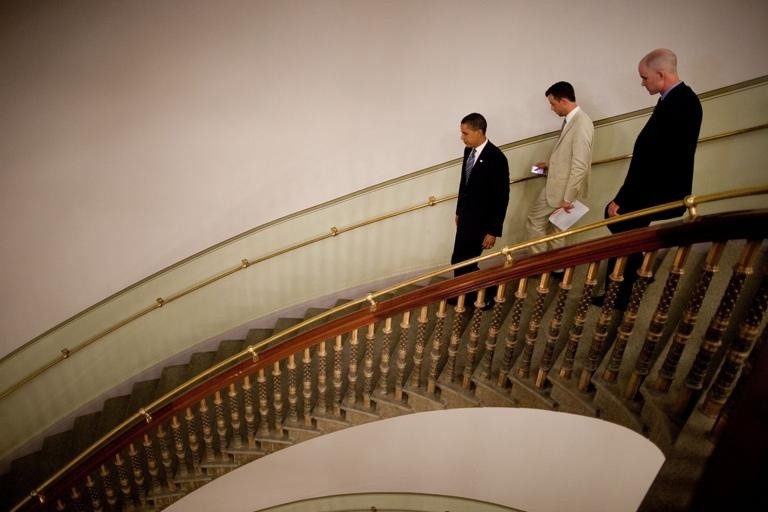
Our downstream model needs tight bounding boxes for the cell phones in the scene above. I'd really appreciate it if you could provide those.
[530,165,546,176]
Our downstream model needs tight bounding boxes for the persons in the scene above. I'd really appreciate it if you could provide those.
[446,112,511,312]
[589,48,701,312]
[524,81,593,279]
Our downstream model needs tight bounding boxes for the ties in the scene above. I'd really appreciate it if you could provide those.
[558,116,567,141]
[463,147,477,181]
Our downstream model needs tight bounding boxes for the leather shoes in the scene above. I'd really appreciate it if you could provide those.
[589,294,629,312]
[448,296,477,306]
[552,271,566,278]
[481,285,497,311]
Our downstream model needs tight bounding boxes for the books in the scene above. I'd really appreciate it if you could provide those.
[548,200,590,232]
